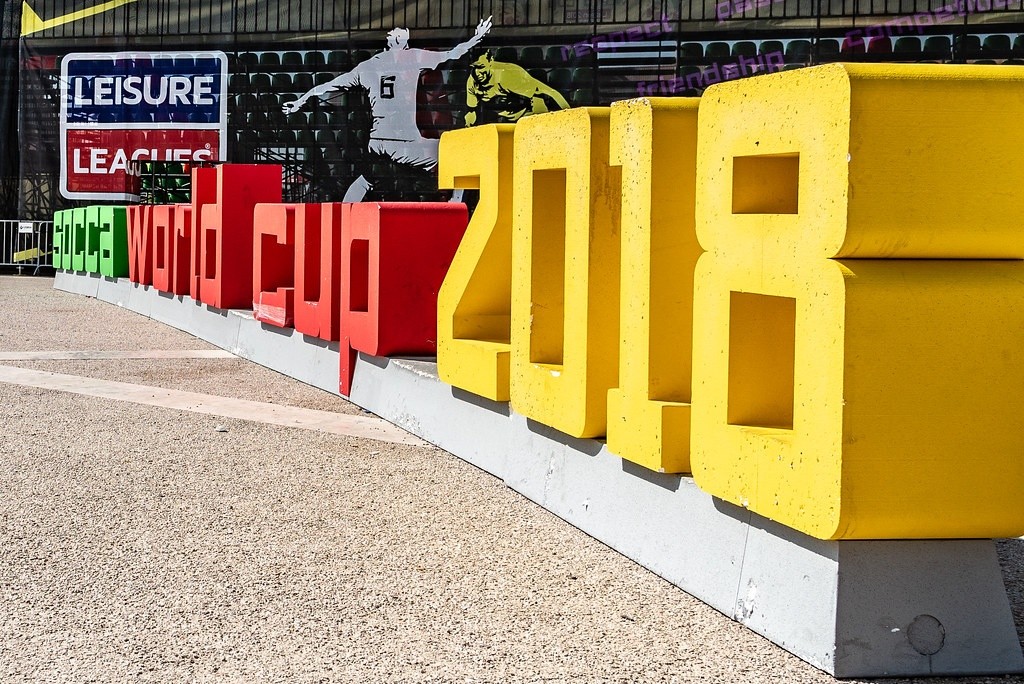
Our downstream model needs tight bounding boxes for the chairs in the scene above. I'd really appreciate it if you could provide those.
[220,33,1024,201]
